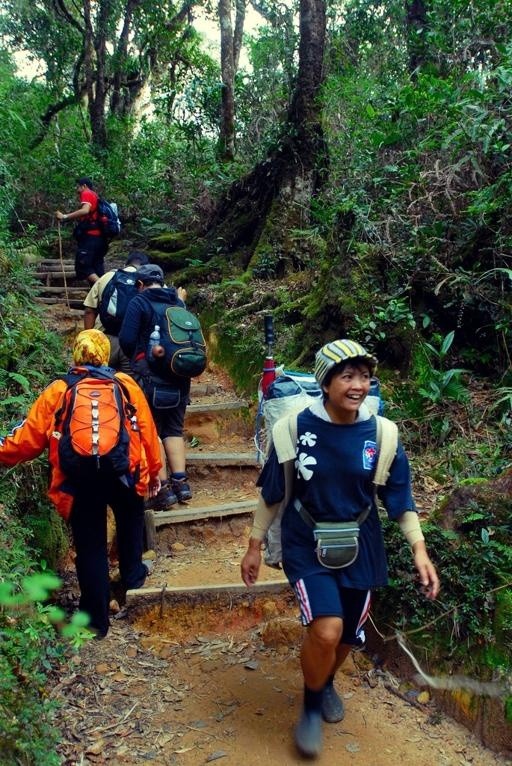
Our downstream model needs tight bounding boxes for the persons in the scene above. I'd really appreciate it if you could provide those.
[1,327,163,644]
[116,263,207,510]
[234,336,441,761]
[81,249,189,374]
[56,171,125,286]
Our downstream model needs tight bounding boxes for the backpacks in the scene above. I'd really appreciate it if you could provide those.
[97,267,138,333]
[55,366,136,483]
[254,370,400,571]
[137,294,208,382]
[86,190,122,238]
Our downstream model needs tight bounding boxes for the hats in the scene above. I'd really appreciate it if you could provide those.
[133,263,165,287]
[313,339,380,388]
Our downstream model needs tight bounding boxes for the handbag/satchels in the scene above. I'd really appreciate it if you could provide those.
[313,522,360,569]
[143,377,181,409]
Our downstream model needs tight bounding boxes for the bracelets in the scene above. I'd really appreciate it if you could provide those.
[63,213,68,221]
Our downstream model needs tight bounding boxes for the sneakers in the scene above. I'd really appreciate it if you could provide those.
[170,477,192,502]
[143,480,178,511]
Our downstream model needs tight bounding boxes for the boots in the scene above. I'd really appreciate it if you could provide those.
[294,682,324,757]
[322,672,346,724]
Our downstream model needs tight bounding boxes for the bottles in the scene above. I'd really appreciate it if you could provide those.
[146,325,161,357]
[260,356,276,394]
